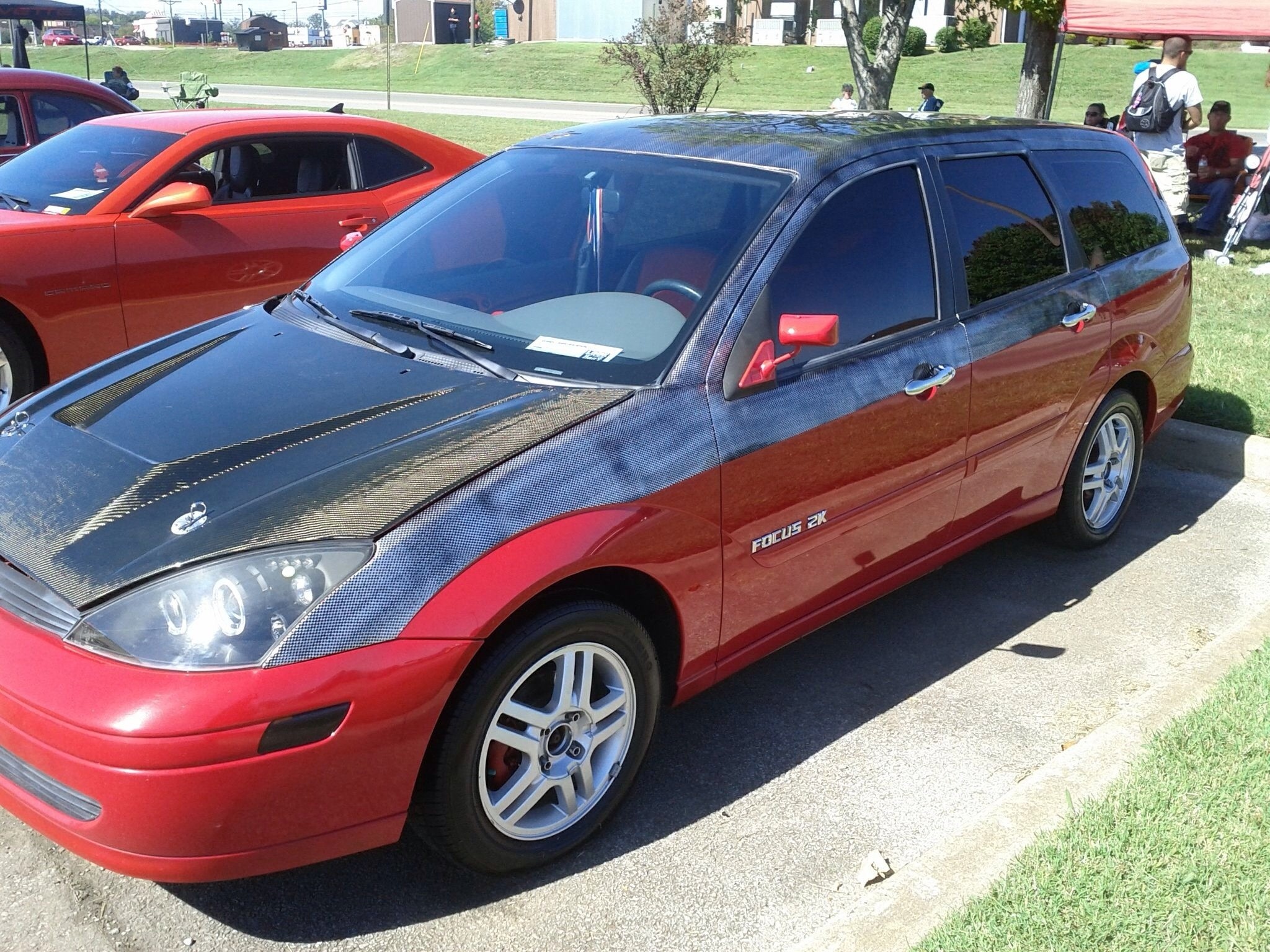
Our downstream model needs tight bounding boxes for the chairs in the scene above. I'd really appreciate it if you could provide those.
[161,71,219,109]
[1189,135,1253,233]
[610,181,730,341]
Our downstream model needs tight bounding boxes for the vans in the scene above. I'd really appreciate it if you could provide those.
[0,115,1194,885]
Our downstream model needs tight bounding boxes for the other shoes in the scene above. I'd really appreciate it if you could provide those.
[1177,221,1193,235]
[1195,228,1210,237]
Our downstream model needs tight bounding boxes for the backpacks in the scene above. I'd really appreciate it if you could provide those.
[1125,66,1185,133]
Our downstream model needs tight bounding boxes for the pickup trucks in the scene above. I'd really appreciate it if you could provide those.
[82,36,107,46]
[114,35,141,46]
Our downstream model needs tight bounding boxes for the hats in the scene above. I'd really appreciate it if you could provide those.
[917,83,935,91]
[1209,100,1231,112]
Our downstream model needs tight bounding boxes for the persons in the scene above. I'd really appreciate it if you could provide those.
[918,83,937,112]
[829,83,859,110]
[469,10,481,44]
[1247,40,1270,275]
[1083,103,1107,126]
[1175,101,1245,236]
[1121,35,1202,227]
[109,66,134,89]
[448,6,461,44]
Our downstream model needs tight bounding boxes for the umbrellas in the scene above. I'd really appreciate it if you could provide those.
[12,19,30,70]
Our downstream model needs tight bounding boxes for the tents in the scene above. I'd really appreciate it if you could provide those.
[0,0,90,81]
[1044,0,1270,121]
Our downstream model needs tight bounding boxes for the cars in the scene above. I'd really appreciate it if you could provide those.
[0,68,143,165]
[0,103,507,417]
[41,29,82,47]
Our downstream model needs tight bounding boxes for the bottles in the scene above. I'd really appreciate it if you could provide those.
[1198,154,1208,175]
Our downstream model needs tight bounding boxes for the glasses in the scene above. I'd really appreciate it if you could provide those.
[1085,111,1102,117]
[1178,50,1193,55]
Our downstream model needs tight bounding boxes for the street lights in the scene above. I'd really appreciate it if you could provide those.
[237,4,243,21]
[292,2,298,27]
[318,9,325,32]
